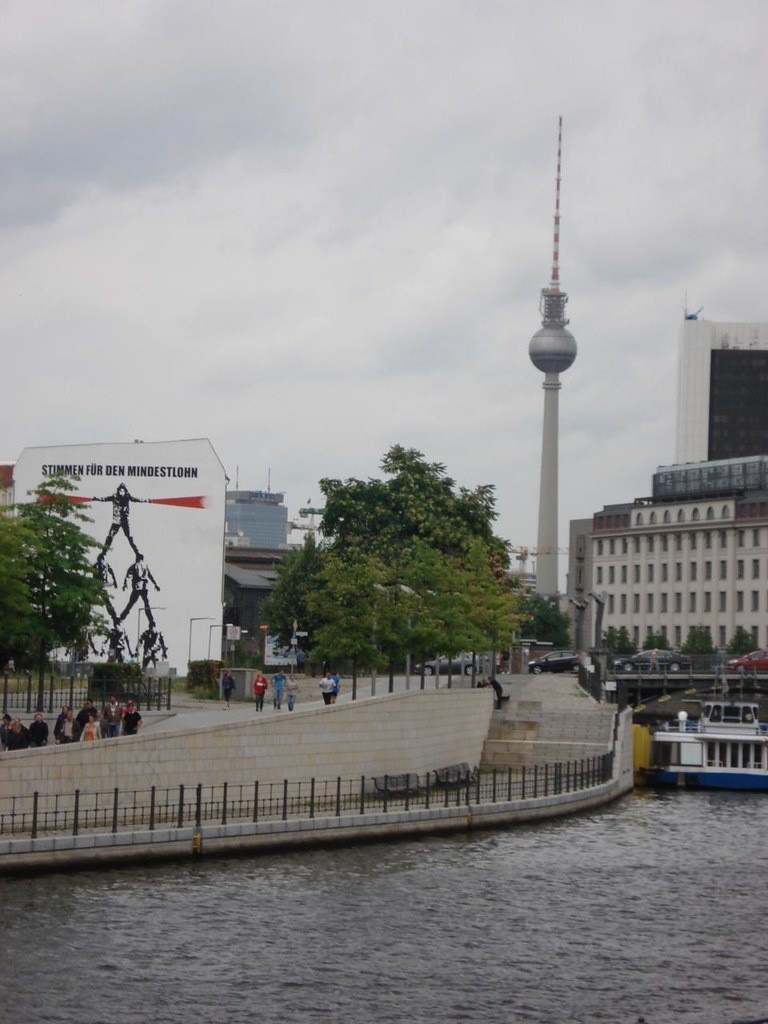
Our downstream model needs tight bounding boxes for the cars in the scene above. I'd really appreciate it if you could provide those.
[528,650,582,675]
[614,648,692,673]
[414,652,481,676]
[726,650,768,674]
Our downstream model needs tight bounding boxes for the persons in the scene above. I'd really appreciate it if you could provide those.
[123,697,138,715]
[27,713,49,748]
[8,655,16,678]
[296,647,306,674]
[648,648,660,675]
[59,710,81,744]
[310,661,318,678]
[103,695,125,738]
[5,720,29,751]
[0,714,12,752]
[13,717,30,749]
[253,670,269,712]
[78,711,102,743]
[501,648,511,675]
[54,705,70,744]
[487,676,510,710]
[122,703,143,736]
[270,667,287,711]
[94,708,111,739]
[477,682,483,688]
[285,674,299,711]
[320,656,330,678]
[330,670,340,704]
[319,671,336,705]
[221,670,237,710]
[76,698,98,734]
[2,661,9,678]
[482,679,489,688]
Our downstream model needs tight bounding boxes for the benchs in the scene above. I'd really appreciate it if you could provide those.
[434,762,479,789]
[372,773,422,801]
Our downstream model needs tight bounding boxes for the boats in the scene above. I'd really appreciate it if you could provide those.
[639,651,768,794]
